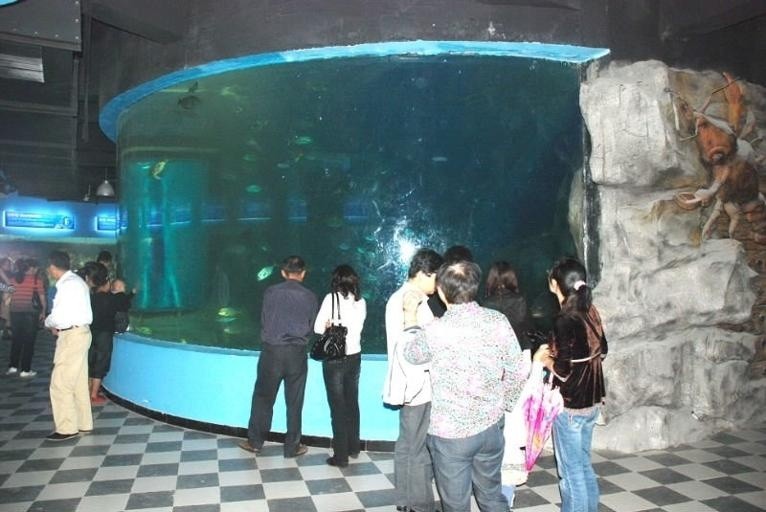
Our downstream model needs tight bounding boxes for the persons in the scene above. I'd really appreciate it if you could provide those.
[313,265,368,469]
[43,252,95,441]
[384,243,607,512]
[237,256,320,456]
[0,251,137,407]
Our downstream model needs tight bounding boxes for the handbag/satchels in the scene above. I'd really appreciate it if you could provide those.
[33,292,43,310]
[596,401,607,426]
[310,326,347,361]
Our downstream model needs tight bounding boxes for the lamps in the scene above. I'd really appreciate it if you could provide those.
[95,167,115,197]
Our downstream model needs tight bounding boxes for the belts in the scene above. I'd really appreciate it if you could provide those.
[56,325,85,332]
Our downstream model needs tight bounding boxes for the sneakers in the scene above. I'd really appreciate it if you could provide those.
[20,370,38,377]
[284,447,308,456]
[6,367,17,375]
[238,439,262,452]
[90,395,109,404]
[45,432,78,442]
[326,452,358,467]
